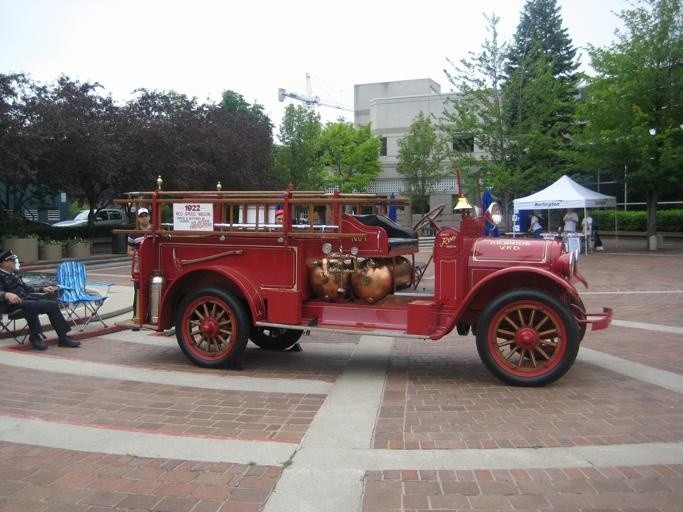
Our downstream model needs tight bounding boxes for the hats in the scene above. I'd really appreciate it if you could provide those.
[138,208,149,216]
[0,249,18,262]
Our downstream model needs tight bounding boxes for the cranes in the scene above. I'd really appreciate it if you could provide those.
[276,73,357,118]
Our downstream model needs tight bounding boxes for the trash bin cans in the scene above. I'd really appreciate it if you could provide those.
[657,233,664,250]
[112,233,128,254]
[649,235,657,251]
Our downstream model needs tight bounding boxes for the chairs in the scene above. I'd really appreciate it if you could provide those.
[55,260,114,332]
[339,213,418,293]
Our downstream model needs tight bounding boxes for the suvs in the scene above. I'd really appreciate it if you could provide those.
[51,207,133,229]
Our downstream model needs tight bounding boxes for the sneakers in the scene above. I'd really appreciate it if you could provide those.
[31,340,47,350]
[58,338,80,347]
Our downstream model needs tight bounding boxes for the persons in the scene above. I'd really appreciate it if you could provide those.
[582,209,594,254]
[126,208,154,331]
[0,249,80,349]
[274,209,284,226]
[561,208,580,254]
[526,212,544,239]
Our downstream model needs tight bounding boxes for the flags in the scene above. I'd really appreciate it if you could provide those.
[482,189,500,240]
[389,194,396,224]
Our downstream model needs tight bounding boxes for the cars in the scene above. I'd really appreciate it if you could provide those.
[128,169,616,387]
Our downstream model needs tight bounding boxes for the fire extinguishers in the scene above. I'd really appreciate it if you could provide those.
[145,270,166,325]
